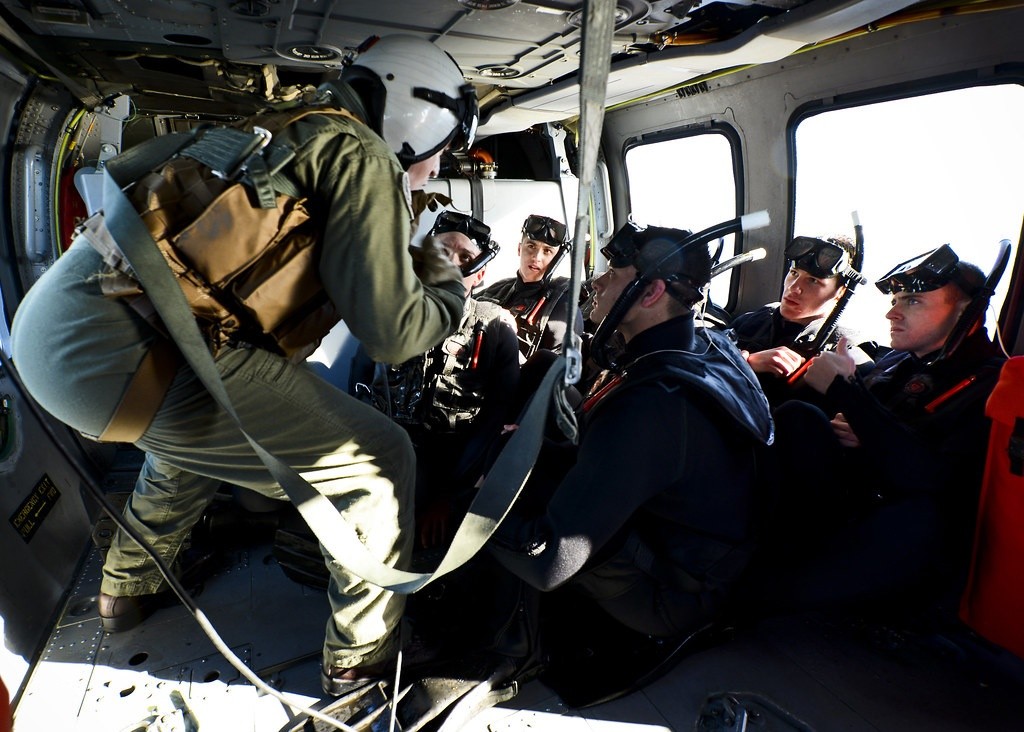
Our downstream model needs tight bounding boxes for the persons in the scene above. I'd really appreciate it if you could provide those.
[10,34,482,701]
[487,221,778,707]
[343,208,1006,640]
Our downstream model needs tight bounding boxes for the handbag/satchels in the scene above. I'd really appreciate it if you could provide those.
[461,554,550,685]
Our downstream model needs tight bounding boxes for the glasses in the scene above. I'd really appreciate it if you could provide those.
[428,210,492,251]
[783,236,848,275]
[599,222,712,313]
[522,214,567,247]
[875,245,977,301]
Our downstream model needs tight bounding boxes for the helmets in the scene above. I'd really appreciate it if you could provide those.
[337,33,479,163]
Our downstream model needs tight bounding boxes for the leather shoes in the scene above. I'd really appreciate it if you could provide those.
[321,616,441,697]
[97,571,199,633]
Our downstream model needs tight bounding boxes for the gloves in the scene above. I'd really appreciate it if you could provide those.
[408,235,467,291]
